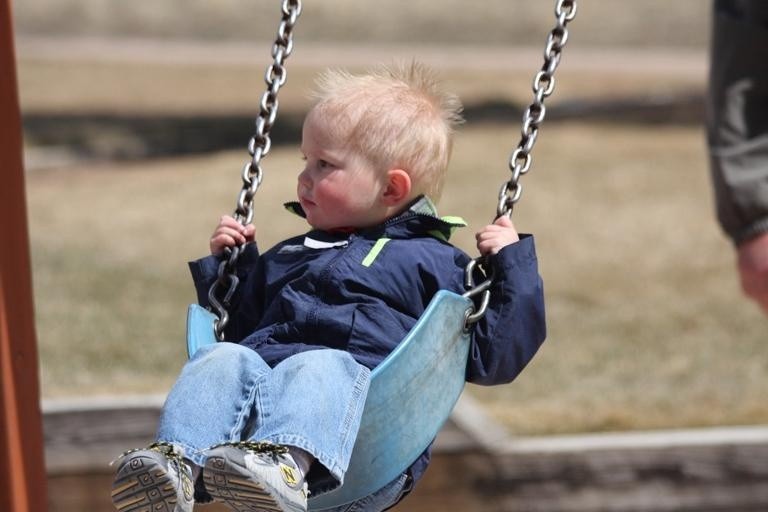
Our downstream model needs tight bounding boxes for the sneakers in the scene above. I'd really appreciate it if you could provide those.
[111,443,195,512]
[203,442,309,512]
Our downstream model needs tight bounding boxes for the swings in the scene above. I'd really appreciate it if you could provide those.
[185,0,576,511]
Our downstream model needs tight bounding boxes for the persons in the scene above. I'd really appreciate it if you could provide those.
[110,74,547,512]
[705,0,767,313]
